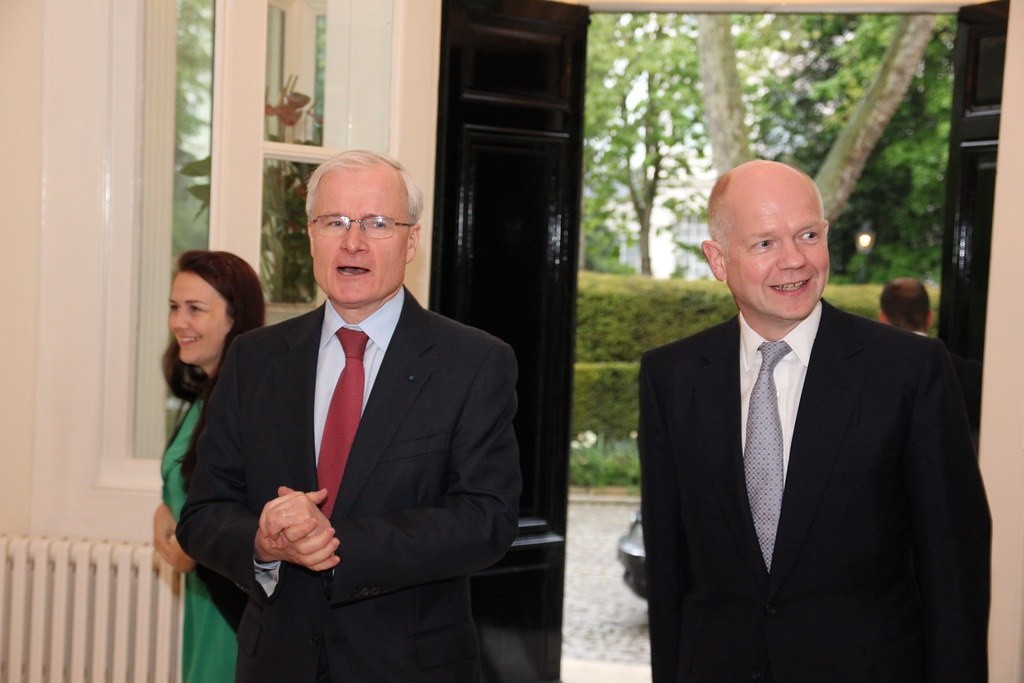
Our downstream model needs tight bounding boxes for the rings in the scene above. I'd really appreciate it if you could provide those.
[282,510,285,517]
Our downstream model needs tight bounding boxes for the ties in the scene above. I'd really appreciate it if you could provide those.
[745,341,792,574]
[316,327,370,520]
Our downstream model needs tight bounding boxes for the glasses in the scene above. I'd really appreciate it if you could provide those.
[309,214,414,239]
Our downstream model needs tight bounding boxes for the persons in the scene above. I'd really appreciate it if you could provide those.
[637,160,993,683]
[879,279,931,336]
[154,250,266,683]
[174,152,522,683]
